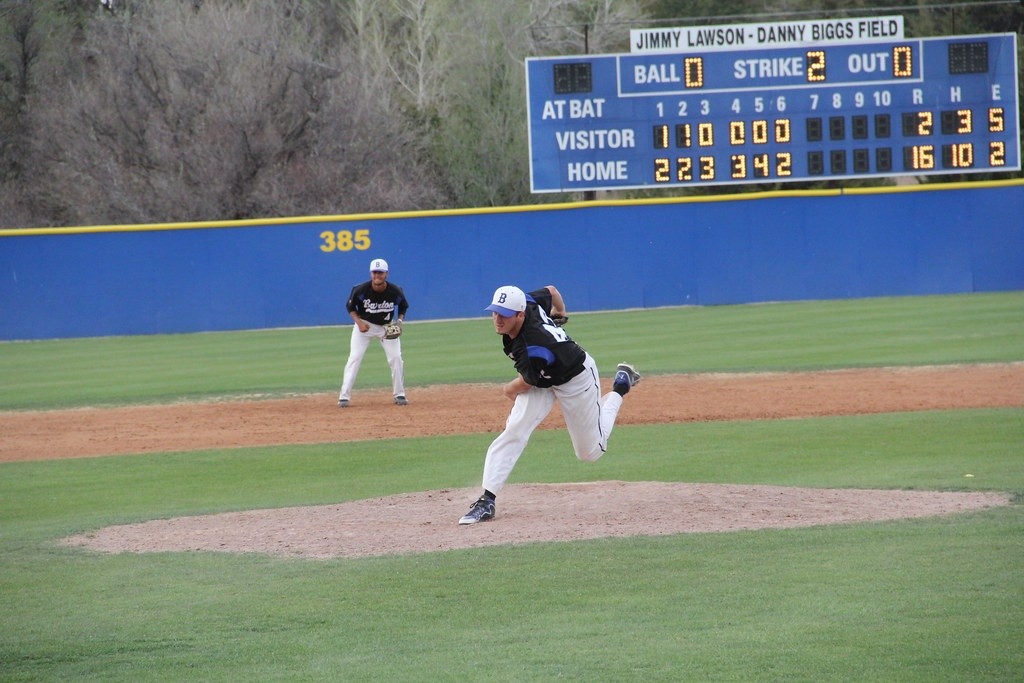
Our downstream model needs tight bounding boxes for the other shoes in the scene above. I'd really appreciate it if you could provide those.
[394,396,408,405]
[337,399,350,408]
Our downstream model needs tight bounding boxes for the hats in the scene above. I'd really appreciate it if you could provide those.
[369,258,388,272]
[484,285,526,318]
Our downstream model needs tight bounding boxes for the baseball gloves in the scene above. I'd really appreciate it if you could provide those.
[550,314,569,327]
[381,319,403,343]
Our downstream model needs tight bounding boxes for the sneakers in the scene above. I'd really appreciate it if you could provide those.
[458,495,496,524]
[614,363,642,391]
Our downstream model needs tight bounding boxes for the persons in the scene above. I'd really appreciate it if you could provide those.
[458,286,640,526]
[337,259,410,407]
